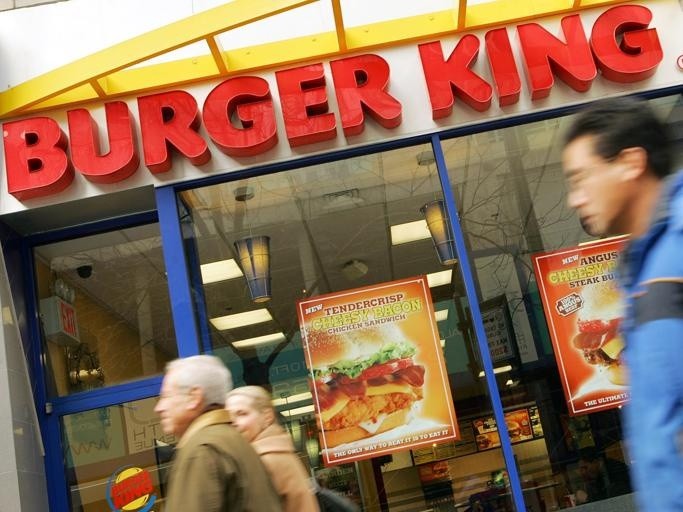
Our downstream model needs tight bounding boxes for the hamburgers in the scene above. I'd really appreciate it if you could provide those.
[302,314,425,450]
[507,423,522,441]
[571,270,628,387]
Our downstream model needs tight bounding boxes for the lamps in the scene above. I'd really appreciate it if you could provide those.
[415,152,460,266]
[40,278,81,347]
[68,343,104,386]
[233,187,272,304]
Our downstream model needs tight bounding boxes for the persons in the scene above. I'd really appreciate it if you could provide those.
[560,91,682,511]
[574,444,631,503]
[228,385,321,512]
[154,354,285,511]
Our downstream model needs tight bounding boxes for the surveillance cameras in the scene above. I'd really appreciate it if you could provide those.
[76,265,93,280]
